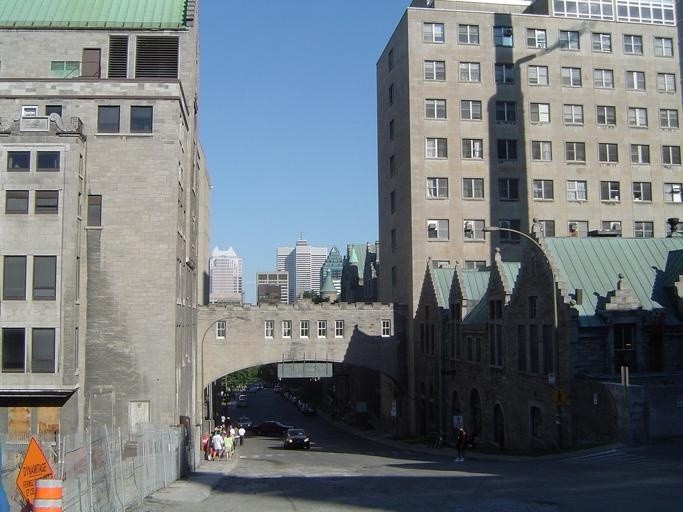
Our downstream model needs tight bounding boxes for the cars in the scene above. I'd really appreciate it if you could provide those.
[237,415,310,449]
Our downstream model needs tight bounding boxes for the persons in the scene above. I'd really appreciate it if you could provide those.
[207,413,246,461]
[447,424,474,462]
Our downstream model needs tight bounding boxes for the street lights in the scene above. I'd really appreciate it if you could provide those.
[482,226,565,452]
[202,316,251,434]
